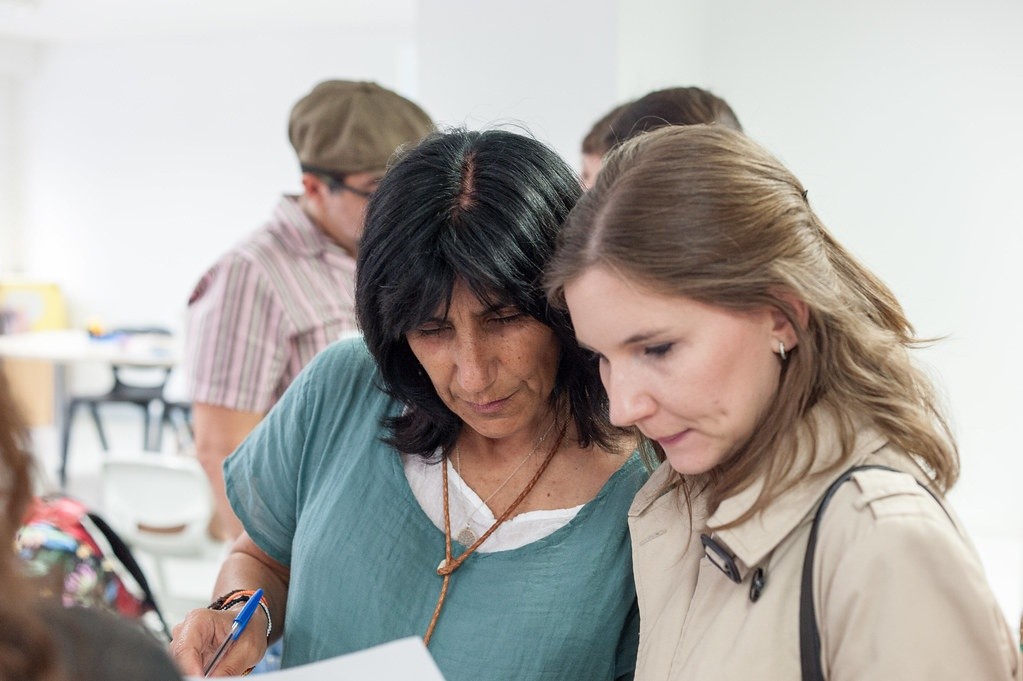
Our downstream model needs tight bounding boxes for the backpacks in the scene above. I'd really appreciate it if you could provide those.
[9,494,173,644]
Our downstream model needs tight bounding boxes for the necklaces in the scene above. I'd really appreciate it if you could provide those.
[456,420,557,545]
[425,413,568,644]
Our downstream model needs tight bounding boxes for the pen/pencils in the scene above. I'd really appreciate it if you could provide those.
[202,588,264,678]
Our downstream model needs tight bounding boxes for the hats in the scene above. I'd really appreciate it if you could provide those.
[288,78,441,175]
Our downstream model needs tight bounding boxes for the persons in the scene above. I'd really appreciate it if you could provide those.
[0,366,185,681]
[579,87,748,193]
[163,79,444,538]
[168,130,663,681]
[543,122,1023,681]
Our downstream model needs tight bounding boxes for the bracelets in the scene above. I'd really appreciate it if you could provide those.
[207,588,274,677]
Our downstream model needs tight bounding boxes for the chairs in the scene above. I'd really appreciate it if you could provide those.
[54,356,181,488]
